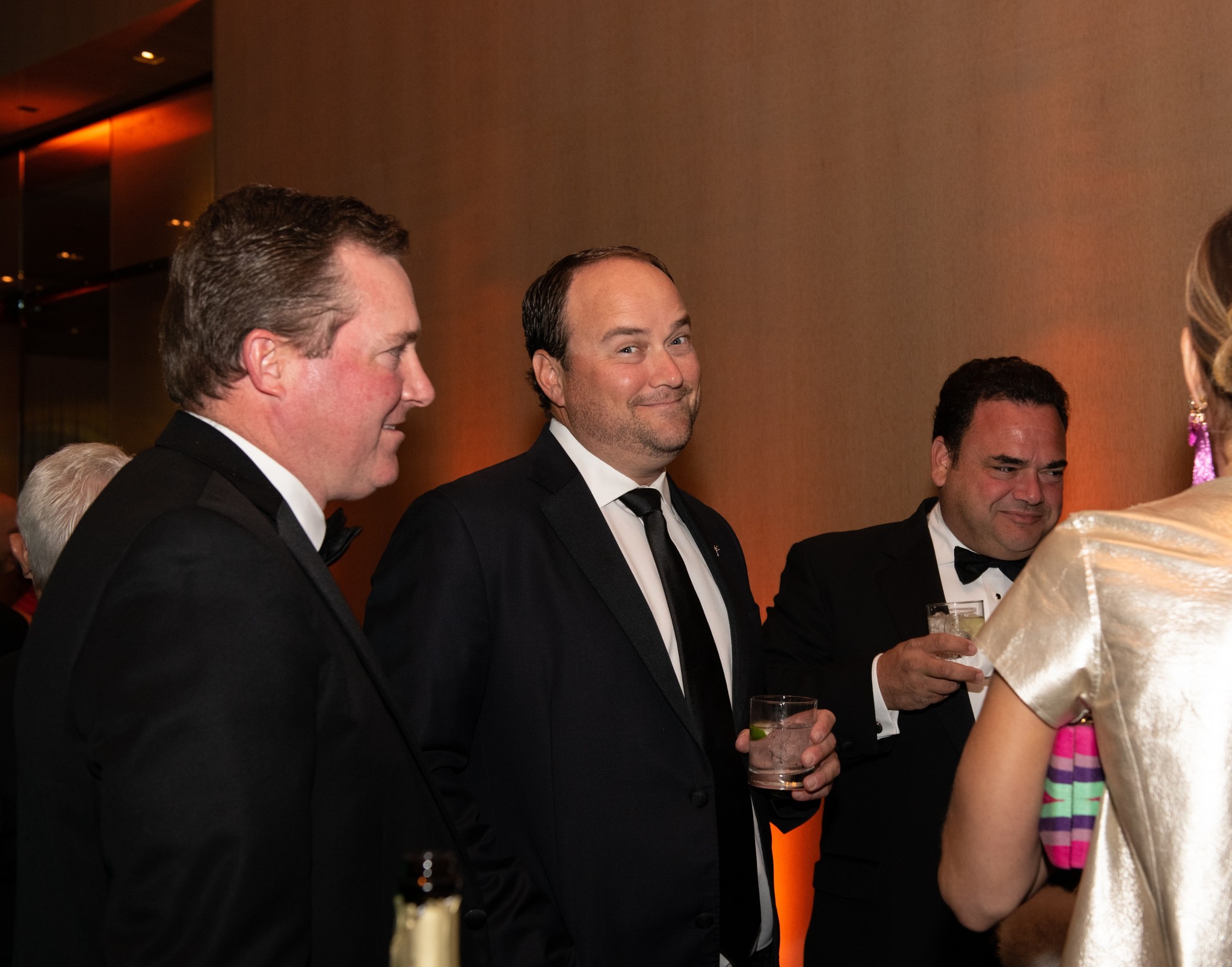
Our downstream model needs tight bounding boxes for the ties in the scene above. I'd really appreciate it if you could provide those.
[618,487,762,967]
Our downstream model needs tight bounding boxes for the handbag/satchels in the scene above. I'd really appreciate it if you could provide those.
[1037,713,1106,890]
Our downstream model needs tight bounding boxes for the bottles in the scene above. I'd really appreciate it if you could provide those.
[388,848,460,967]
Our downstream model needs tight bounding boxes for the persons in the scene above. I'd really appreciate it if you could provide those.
[757,209,1232,967]
[0,185,478,967]
[362,244,842,967]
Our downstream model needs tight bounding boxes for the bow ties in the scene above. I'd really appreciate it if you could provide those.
[954,545,1031,585]
[318,506,362,568]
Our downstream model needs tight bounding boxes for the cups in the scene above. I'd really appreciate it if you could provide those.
[748,694,817,792]
[927,600,991,680]
